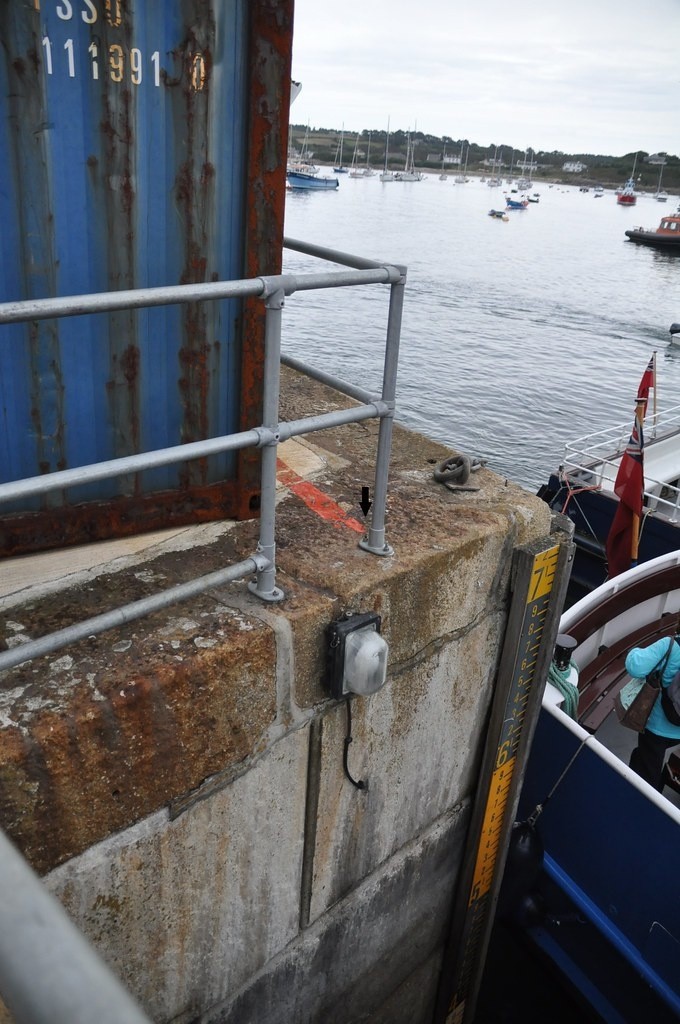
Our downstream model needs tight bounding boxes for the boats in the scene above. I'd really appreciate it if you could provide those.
[505,546,680,1024]
[529,353,680,603]
[618,152,641,205]
[527,196,539,202]
[286,170,340,188]
[658,190,668,203]
[625,201,680,246]
[505,196,528,210]
[580,183,590,191]
[593,185,603,192]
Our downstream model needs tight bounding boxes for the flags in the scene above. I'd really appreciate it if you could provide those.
[606,415,644,580]
[633,357,653,418]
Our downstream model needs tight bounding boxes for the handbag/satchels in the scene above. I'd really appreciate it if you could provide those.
[614,636,674,733]
[661,669,680,727]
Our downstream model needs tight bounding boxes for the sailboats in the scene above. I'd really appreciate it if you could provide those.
[440,140,448,181]
[287,114,377,177]
[395,115,421,182]
[480,144,533,190]
[377,113,395,180]
[455,141,470,183]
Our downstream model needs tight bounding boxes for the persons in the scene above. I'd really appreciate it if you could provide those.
[624,622,680,794]
